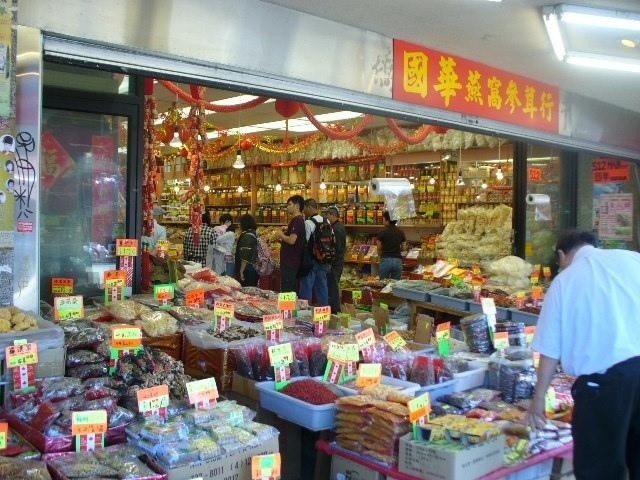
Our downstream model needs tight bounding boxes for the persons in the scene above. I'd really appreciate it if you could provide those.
[324,205,347,313]
[213,213,236,278]
[151,219,167,240]
[376,211,407,281]
[236,213,260,287]
[217,225,239,274]
[276,194,306,296]
[299,197,329,308]
[522,228,640,480]
[183,214,225,277]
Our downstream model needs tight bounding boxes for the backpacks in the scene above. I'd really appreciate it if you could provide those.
[310,217,337,263]
[246,233,275,277]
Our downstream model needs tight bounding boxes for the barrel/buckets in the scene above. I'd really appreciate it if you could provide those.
[208,163,370,224]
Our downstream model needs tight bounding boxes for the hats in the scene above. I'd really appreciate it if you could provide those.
[152,205,167,216]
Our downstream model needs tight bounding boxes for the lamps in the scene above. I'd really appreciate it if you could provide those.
[541,4,640,74]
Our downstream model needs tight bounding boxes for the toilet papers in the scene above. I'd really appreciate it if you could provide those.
[526,193,552,221]
[370,177,417,221]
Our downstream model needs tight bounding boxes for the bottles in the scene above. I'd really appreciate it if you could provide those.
[204,164,442,225]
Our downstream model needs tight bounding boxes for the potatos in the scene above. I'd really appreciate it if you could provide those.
[0,304,39,334]
